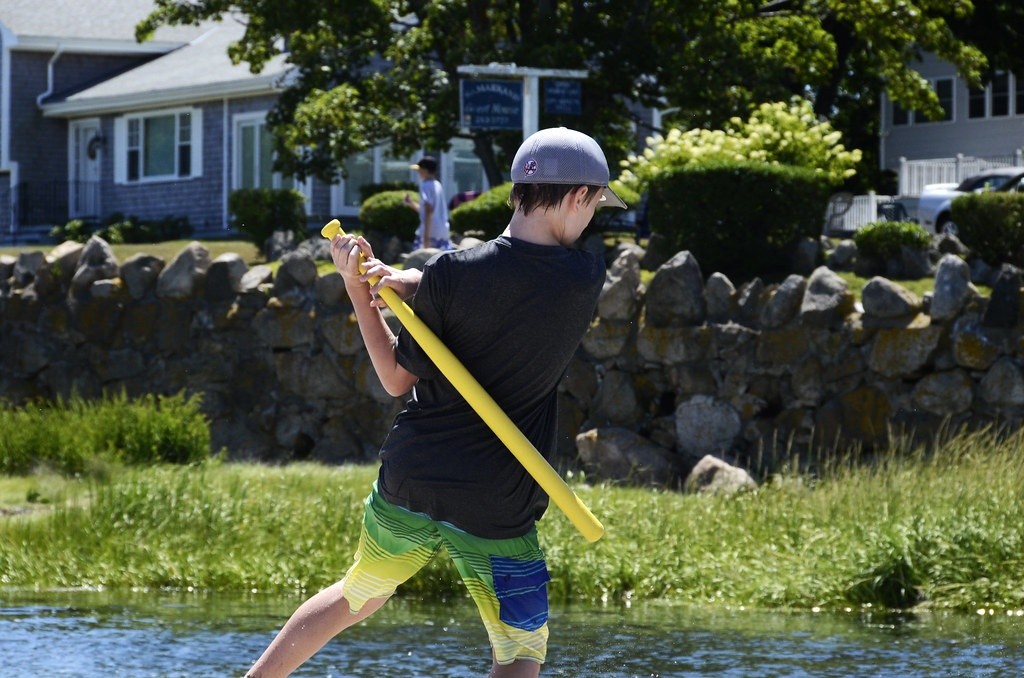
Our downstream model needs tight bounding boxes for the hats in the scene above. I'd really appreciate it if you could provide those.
[510,125,628,210]
[409,157,437,173]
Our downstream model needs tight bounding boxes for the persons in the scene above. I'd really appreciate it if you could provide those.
[413,157,451,252]
[244,127,627,678]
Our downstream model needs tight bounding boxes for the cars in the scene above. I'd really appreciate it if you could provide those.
[921,166,1024,236]
[880,195,919,224]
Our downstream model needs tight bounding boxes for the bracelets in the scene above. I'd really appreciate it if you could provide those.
[422,237,430,243]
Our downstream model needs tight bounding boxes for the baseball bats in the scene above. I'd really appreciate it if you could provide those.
[320,219,606,543]
